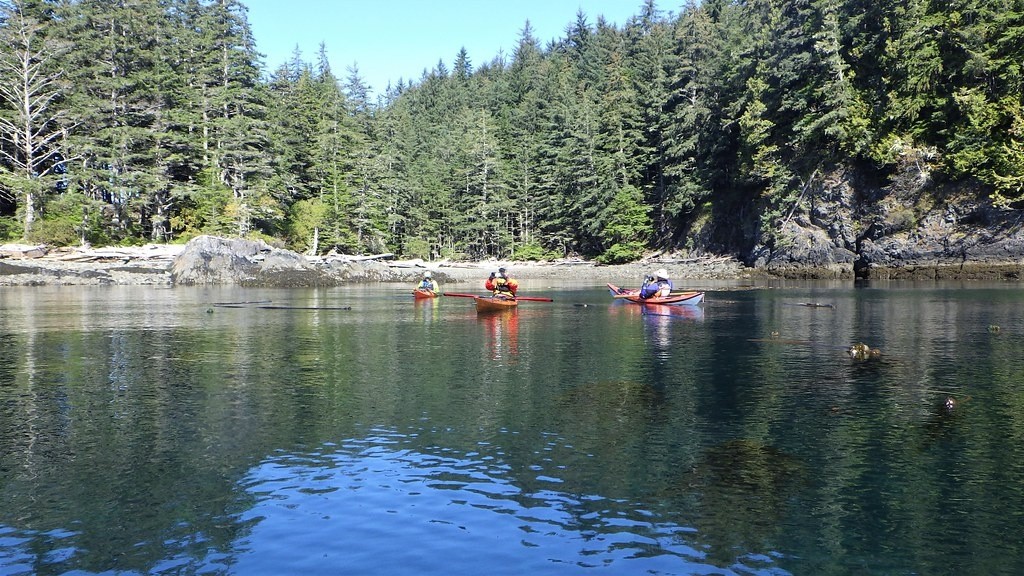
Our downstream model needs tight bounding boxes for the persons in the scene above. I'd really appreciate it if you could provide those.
[484,267,518,301]
[640,266,673,299]
[416,271,440,292]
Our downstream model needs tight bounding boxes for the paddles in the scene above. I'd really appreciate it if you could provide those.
[443,293,553,302]
[614,292,697,299]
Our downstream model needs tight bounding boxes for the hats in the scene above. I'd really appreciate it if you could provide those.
[499,268,505,273]
[657,269,669,278]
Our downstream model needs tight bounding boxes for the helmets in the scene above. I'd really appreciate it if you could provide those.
[424,271,431,277]
[649,276,654,281]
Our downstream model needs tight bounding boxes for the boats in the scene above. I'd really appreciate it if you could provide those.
[474,296,517,313]
[414,288,438,299]
[606,282,705,306]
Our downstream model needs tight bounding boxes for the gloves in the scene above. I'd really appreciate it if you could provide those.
[491,272,496,278]
